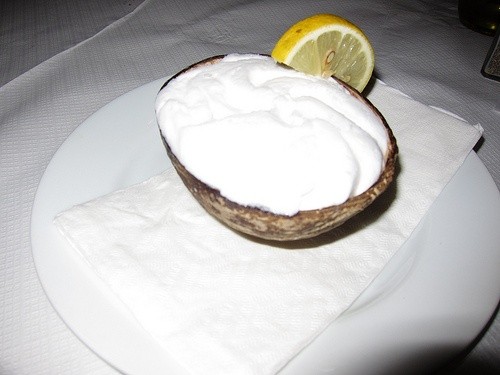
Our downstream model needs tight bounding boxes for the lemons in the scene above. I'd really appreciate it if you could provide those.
[272,14,375,94]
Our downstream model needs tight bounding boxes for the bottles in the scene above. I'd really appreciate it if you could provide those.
[458,0,500,36]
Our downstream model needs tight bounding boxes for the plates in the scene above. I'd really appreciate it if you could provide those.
[31,73,500,375]
